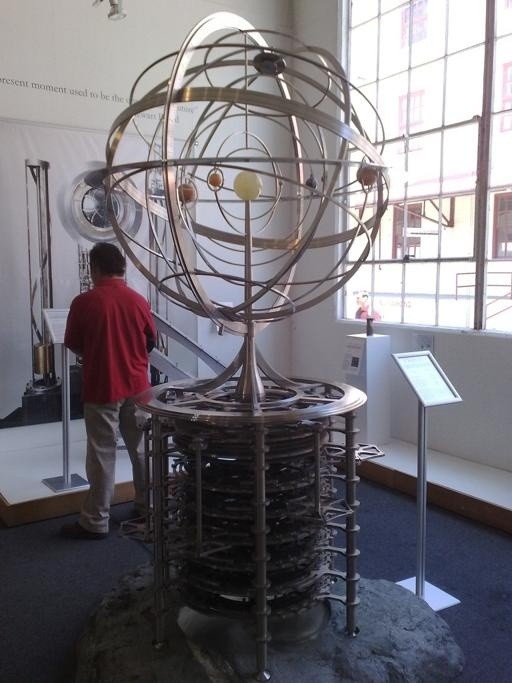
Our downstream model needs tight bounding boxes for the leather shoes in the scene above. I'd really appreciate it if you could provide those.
[64,524,107,539]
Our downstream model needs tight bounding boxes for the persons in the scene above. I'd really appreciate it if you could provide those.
[62,242,158,542]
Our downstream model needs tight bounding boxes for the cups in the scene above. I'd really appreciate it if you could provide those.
[366,317,375,336]
